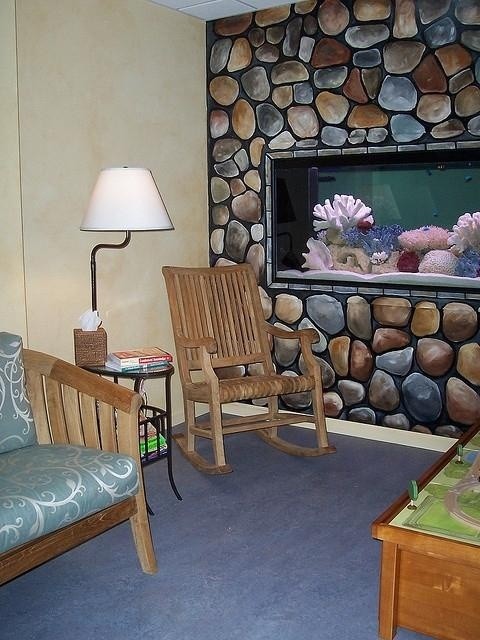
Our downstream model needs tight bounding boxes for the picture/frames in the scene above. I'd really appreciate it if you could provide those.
[265,141,480,299]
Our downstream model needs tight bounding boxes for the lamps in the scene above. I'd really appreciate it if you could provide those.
[80,165,174,309]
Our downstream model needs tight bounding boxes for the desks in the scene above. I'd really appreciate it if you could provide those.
[81,362,182,515]
[371,418,480,640]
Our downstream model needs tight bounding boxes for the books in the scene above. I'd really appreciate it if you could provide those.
[137,408,167,463]
[105,346,173,373]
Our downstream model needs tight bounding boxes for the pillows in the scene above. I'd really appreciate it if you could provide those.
[0,332,37,454]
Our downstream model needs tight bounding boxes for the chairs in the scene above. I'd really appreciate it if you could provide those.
[161,263,337,475]
[0,348,158,584]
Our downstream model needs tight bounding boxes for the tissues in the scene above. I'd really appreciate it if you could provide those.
[73,308,108,366]
